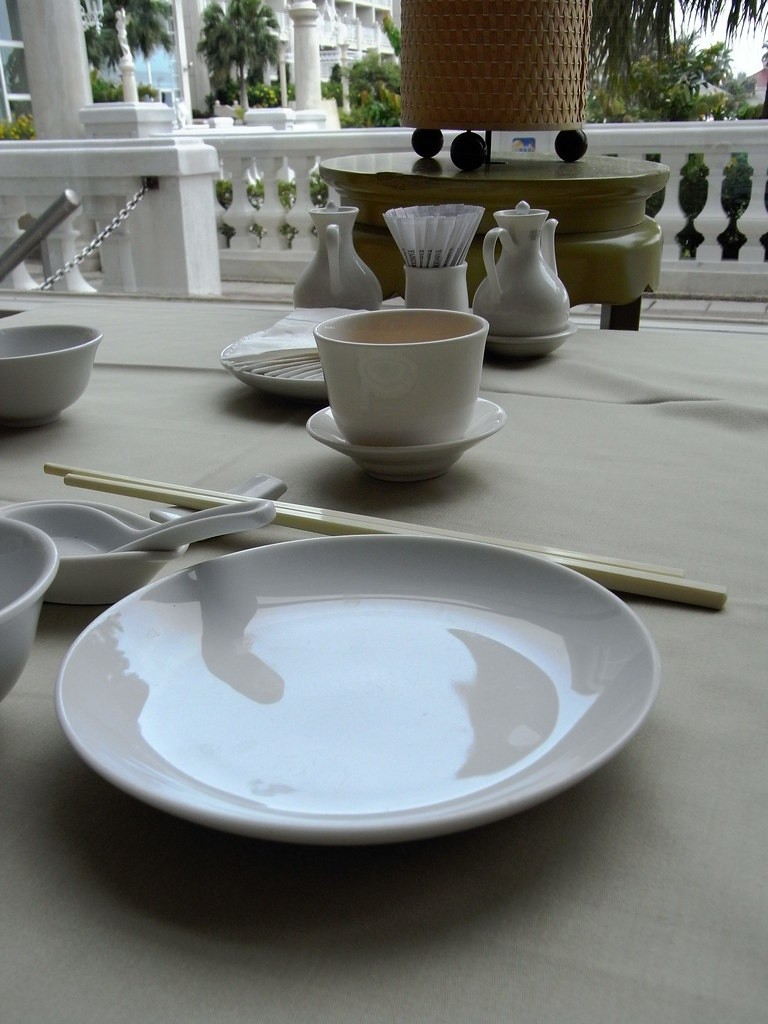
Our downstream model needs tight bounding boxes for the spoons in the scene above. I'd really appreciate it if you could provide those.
[4,500,276,555]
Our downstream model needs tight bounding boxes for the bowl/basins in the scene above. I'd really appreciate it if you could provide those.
[0,499,190,604]
[0,323,105,428]
[0,516,60,703]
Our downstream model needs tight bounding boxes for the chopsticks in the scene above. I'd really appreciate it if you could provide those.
[42,460,729,609]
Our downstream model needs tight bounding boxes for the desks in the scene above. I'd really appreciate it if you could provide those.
[319,150,670,330]
[0,287,768,1024]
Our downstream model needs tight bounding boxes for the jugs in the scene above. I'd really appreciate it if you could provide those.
[472,200,570,337]
[290,201,383,311]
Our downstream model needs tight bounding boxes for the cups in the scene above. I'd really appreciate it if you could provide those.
[313,308,490,446]
[403,262,468,313]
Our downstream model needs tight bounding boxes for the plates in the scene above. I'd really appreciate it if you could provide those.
[54,534,662,847]
[487,321,578,361]
[219,342,329,400]
[306,397,507,481]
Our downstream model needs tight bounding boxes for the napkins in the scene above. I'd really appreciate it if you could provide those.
[224,307,371,383]
